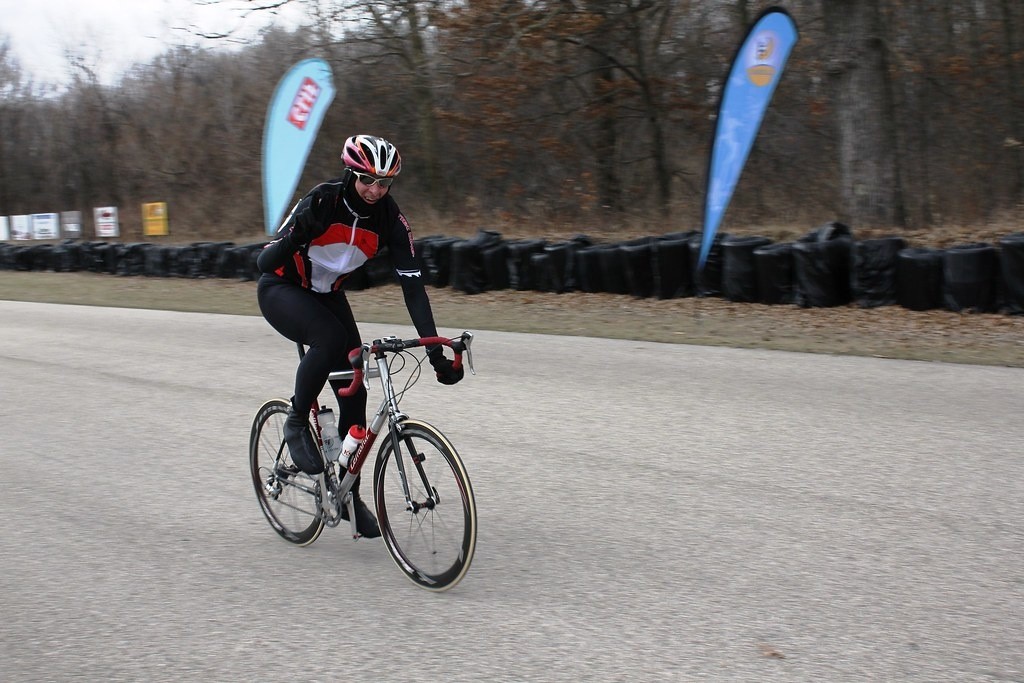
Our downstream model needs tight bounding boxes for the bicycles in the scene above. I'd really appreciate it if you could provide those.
[248,330,476,590]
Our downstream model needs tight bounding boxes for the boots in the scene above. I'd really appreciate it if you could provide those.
[283,400,325,475]
[339,466,378,535]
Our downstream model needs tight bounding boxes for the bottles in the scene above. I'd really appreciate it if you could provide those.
[316,404,342,461]
[337,425,366,468]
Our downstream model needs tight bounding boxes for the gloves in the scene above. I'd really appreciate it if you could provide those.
[431,360,464,384]
[291,190,335,247]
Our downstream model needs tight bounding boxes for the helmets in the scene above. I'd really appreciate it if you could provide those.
[340,134,402,177]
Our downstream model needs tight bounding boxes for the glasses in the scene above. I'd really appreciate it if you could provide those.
[345,166,394,188]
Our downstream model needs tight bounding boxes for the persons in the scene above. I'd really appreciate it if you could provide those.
[256,134,465,539]
